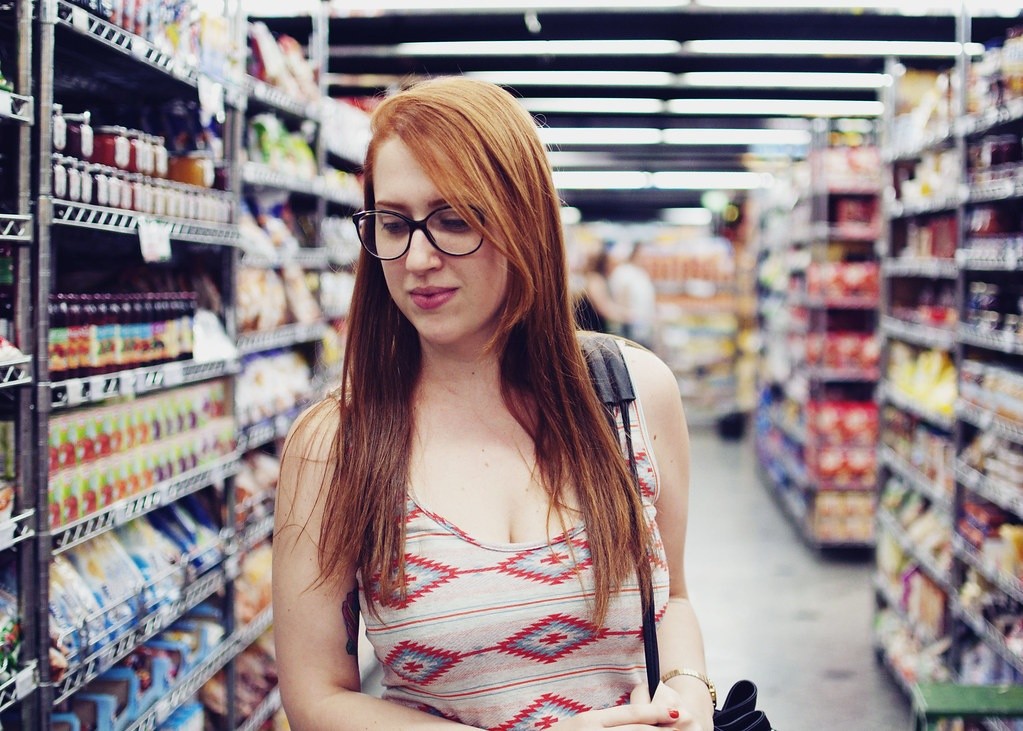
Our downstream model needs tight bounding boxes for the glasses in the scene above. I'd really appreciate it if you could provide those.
[352,204,490,261]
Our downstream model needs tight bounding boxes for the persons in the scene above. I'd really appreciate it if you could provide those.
[572,239,658,350]
[274,74,717,731]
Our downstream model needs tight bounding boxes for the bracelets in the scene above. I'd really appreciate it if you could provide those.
[660,669,716,708]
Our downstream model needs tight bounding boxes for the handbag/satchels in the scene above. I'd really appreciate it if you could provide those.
[582,329,771,731]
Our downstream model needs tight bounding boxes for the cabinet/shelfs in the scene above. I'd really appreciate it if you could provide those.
[595,1,1023,731]
[0,0,378,731]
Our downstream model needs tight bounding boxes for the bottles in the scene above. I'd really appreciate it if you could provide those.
[0,291,204,381]
